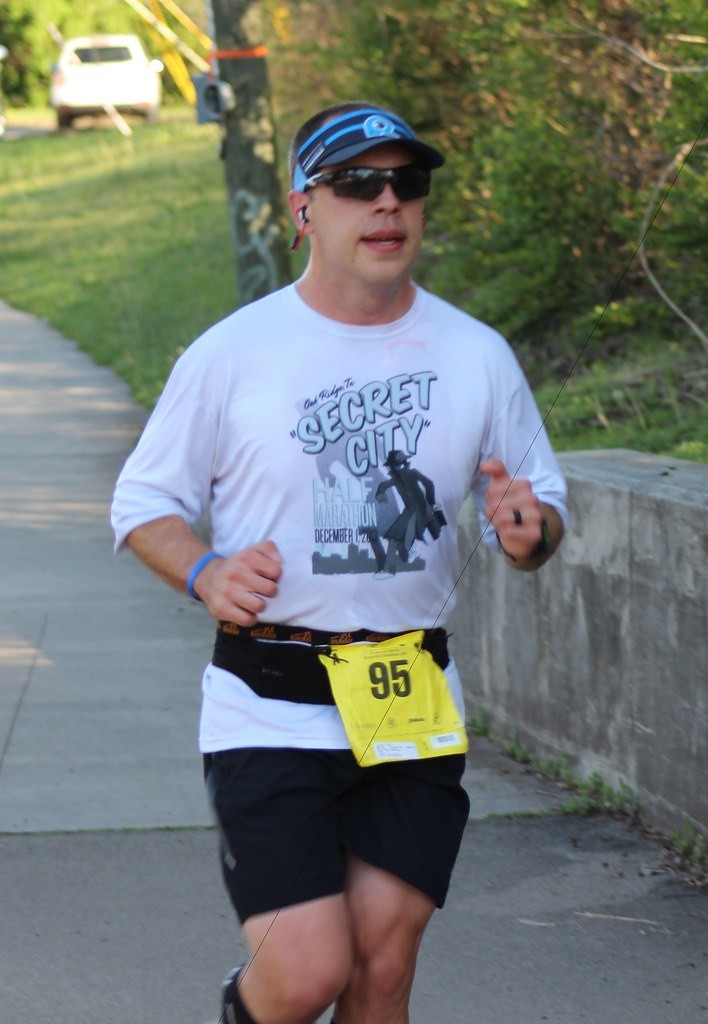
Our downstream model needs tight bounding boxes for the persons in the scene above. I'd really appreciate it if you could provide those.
[110,104,569,1024]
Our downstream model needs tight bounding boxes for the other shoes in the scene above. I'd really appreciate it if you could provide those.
[216,964,247,1024]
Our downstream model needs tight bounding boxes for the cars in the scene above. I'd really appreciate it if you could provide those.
[48,34,165,127]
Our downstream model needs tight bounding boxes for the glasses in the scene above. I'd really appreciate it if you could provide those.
[303,162,430,201]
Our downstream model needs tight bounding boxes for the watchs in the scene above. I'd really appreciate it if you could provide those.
[495,519,551,563]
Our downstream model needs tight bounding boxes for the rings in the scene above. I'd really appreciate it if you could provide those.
[513,510,522,524]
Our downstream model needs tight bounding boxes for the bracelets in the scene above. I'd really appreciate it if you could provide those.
[186,551,224,601]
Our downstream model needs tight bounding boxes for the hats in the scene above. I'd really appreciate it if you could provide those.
[294,107,445,194]
